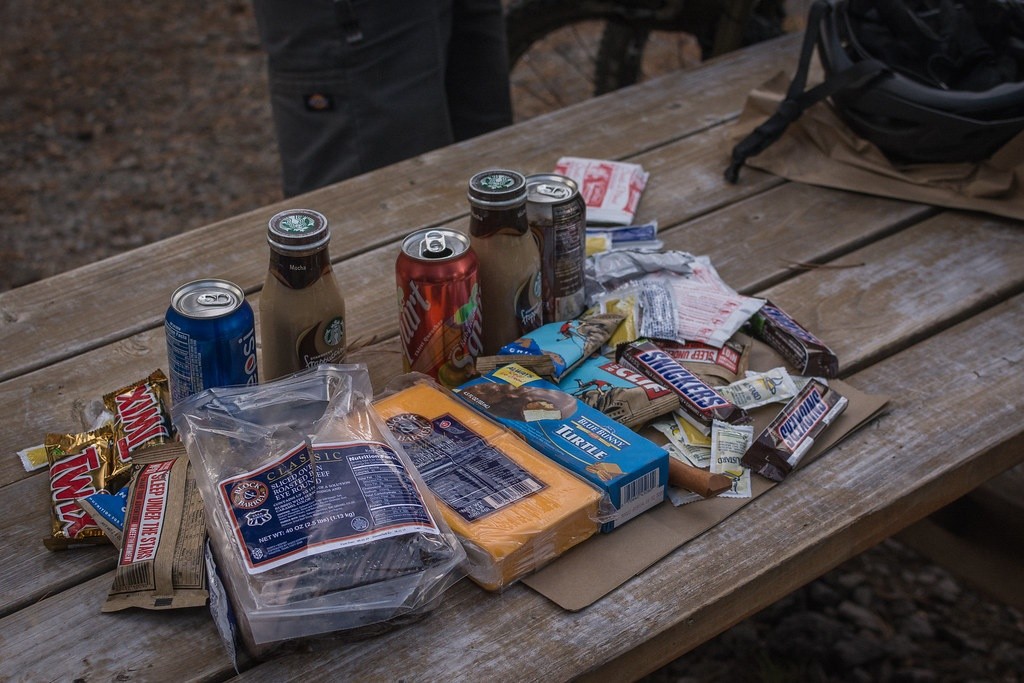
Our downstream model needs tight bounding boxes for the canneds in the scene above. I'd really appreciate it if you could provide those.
[164,278,260,407]
[521,172,586,323]
[395,228,483,389]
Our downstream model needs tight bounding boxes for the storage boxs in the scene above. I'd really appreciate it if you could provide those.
[310,362,668,596]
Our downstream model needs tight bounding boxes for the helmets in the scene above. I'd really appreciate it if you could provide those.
[815,0,1024,165]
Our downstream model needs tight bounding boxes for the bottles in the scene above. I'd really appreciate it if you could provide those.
[465,169,544,357]
[258,208,346,380]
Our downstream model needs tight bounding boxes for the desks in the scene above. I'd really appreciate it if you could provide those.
[0,31,1024,682]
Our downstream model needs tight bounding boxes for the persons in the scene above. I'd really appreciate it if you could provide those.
[249,0,512,200]
